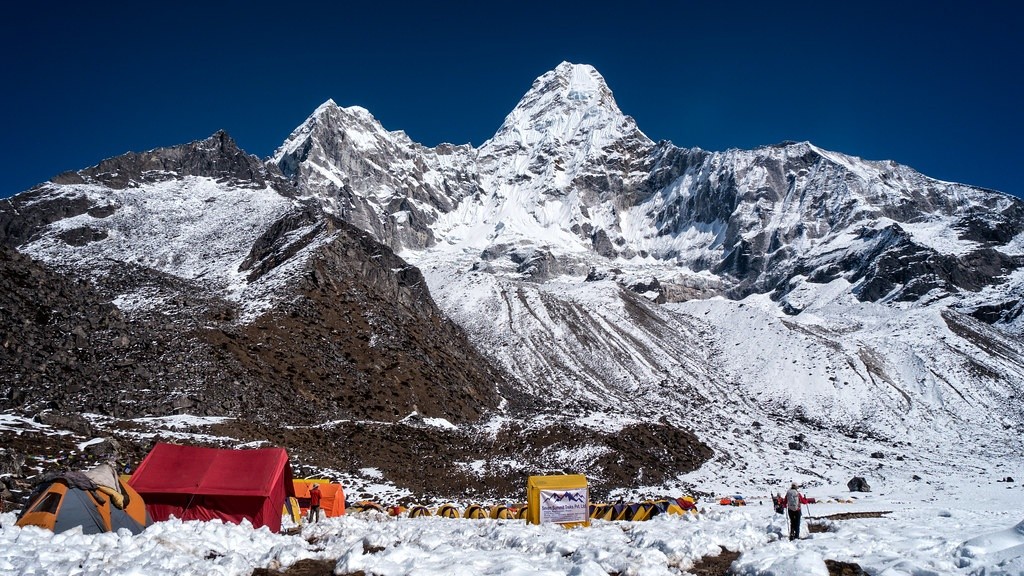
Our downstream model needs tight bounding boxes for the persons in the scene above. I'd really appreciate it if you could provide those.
[771,492,785,516]
[308,483,322,523]
[781,482,809,540]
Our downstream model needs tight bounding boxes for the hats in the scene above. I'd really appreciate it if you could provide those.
[792,482,798,489]
[313,483,319,488]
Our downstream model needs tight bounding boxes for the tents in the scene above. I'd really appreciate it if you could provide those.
[463,504,488,522]
[526,474,590,530]
[434,502,462,521]
[408,506,432,520]
[360,502,386,520]
[489,504,515,521]
[720,495,747,507]
[14,462,155,538]
[591,494,699,527]
[288,482,345,518]
[390,503,407,519]
[515,506,528,520]
[352,499,369,521]
[125,440,301,531]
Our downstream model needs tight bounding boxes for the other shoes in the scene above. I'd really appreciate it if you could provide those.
[790,534,793,541]
